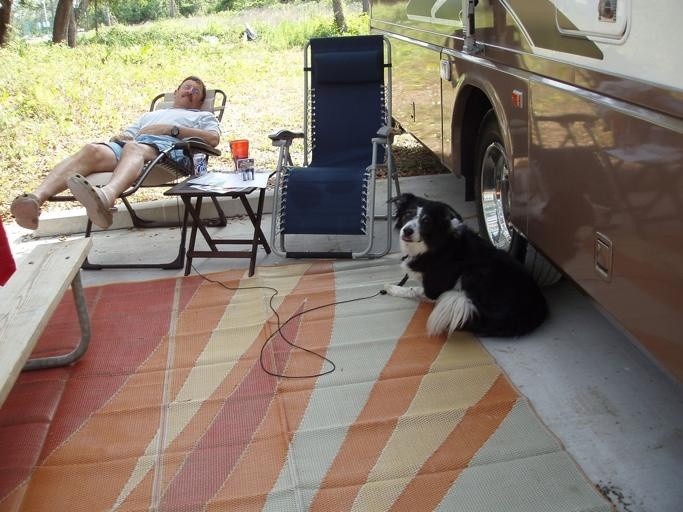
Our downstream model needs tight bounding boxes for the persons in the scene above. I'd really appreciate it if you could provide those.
[8,74,221,231]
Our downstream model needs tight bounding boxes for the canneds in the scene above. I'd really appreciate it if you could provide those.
[192,153,207,177]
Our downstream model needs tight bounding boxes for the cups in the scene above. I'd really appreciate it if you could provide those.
[228,138,248,171]
[235,158,255,181]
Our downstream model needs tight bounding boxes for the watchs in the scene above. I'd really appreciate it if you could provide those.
[169,124,179,139]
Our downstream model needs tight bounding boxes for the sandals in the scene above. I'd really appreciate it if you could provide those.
[65,173,117,230]
[10,193,41,230]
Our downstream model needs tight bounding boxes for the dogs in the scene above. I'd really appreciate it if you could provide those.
[383,191,551,340]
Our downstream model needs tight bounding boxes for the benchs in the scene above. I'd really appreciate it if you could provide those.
[1,237,99,420]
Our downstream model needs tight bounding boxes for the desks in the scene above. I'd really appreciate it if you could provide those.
[161,168,279,282]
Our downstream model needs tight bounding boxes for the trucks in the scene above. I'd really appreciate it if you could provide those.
[369,0,680,394]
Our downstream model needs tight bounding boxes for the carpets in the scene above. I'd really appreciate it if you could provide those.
[1,252,621,511]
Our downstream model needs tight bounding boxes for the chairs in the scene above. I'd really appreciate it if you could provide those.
[43,89,231,271]
[436,20,601,244]
[269,35,406,260]
